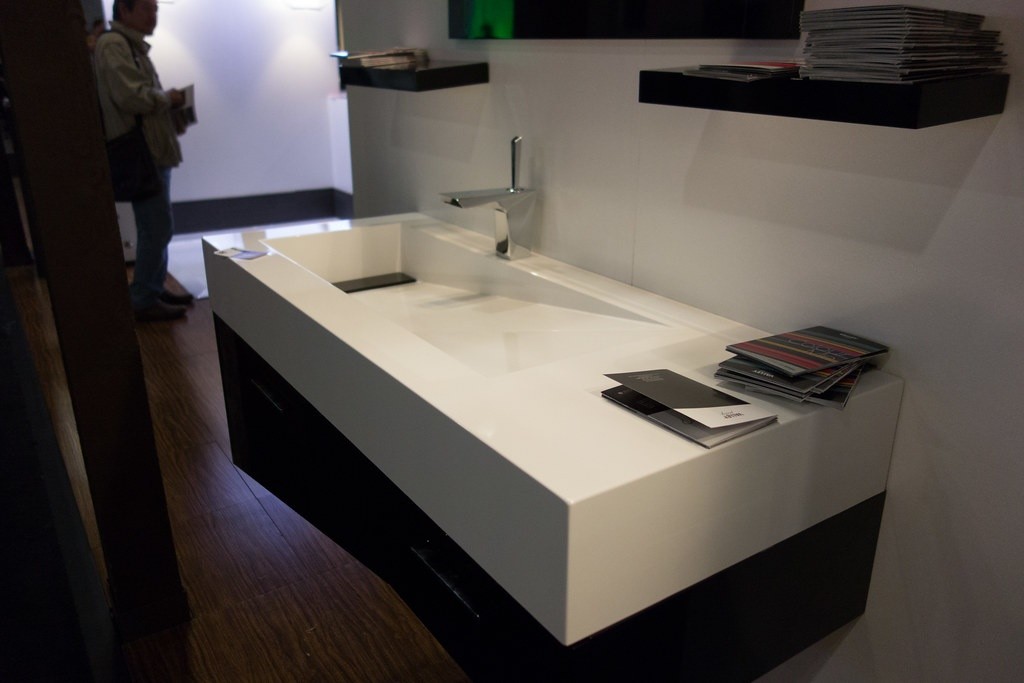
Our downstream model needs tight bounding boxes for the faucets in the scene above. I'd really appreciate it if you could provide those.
[436,136,536,260]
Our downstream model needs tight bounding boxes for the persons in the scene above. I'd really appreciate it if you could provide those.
[94,0,196,325]
[87,20,106,63]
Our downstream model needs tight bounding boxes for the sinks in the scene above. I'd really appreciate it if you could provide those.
[258,221,707,382]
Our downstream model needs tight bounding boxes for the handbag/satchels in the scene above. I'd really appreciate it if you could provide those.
[106,127,163,205]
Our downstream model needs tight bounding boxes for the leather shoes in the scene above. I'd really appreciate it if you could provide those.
[153,286,195,308]
[131,292,187,326]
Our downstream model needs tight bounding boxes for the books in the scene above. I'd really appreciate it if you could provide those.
[330,47,430,68]
[794,5,1012,85]
[713,325,891,411]
[684,60,801,85]
[601,369,779,449]
